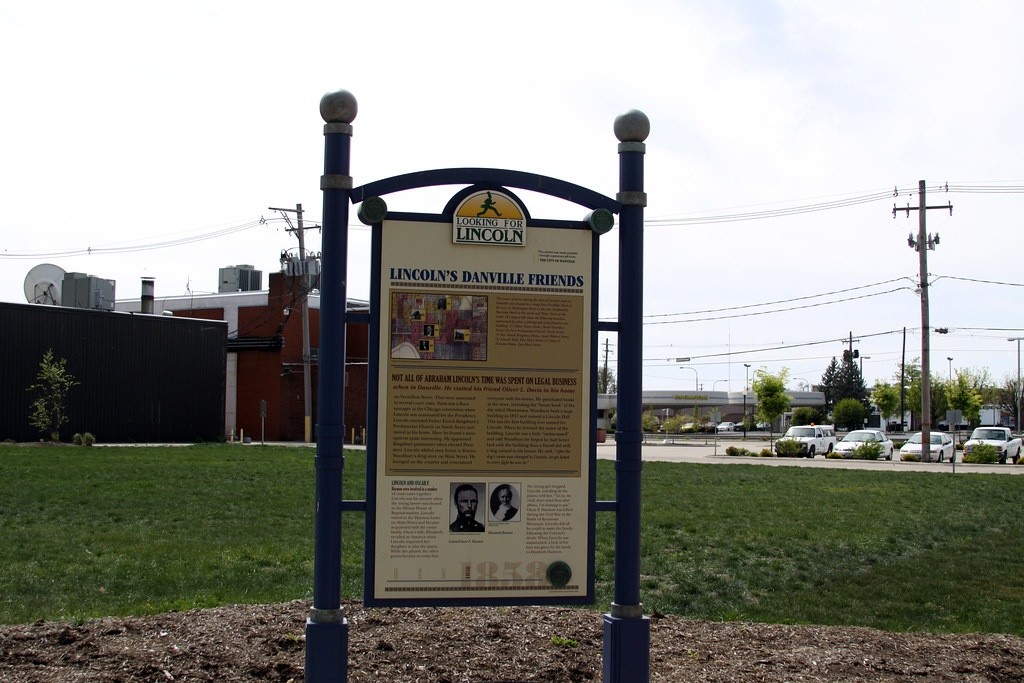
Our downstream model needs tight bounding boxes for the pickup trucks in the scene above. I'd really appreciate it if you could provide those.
[963,427,1022,464]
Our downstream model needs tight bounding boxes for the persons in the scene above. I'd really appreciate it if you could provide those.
[426,325,433,336]
[449,485,485,532]
[492,489,518,521]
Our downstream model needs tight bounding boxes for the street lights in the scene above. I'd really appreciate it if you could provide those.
[680,367,698,404]
[794,378,810,392]
[1007,337,1024,434]
[662,409,668,418]
[713,380,727,422]
[744,364,751,411]
[947,357,954,386]
[861,357,873,380]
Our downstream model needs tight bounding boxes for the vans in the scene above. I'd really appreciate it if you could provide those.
[775,425,836,458]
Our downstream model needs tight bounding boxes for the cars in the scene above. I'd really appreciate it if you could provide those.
[681,422,718,434]
[900,432,956,462]
[717,422,735,431]
[756,422,771,428]
[832,430,894,461]
[734,422,747,432]
